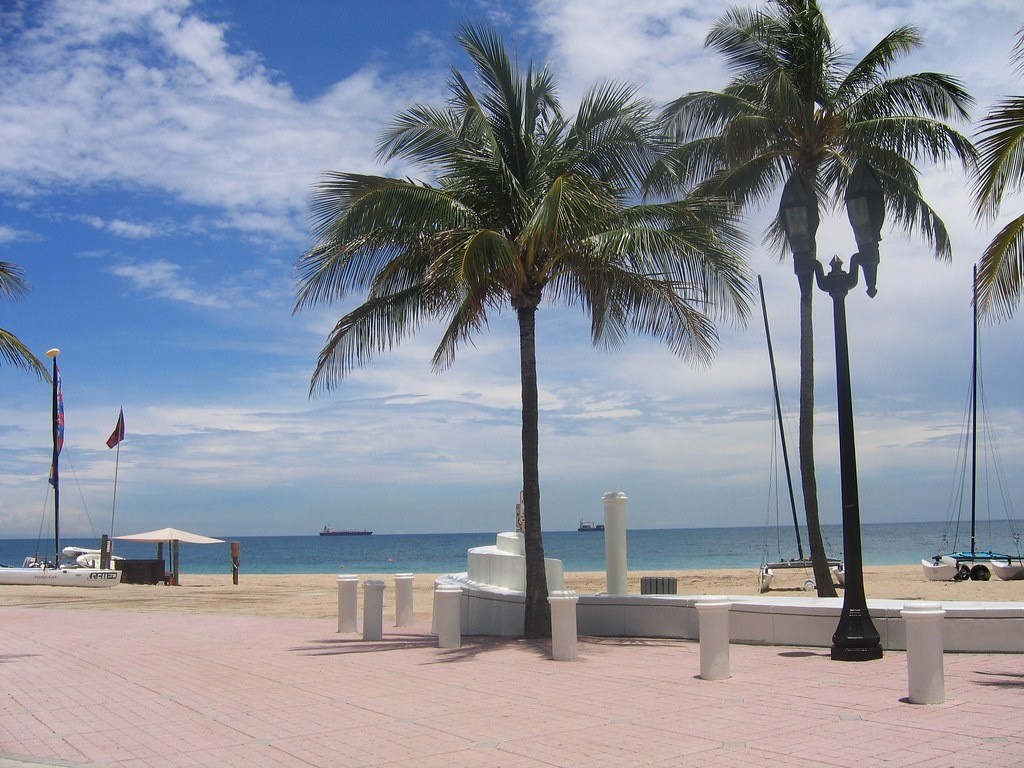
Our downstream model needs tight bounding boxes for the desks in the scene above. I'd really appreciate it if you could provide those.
[114,558,165,584]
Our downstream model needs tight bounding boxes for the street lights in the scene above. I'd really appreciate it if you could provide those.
[777,155,885,664]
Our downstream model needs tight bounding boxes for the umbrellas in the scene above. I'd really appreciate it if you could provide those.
[107,527,226,573]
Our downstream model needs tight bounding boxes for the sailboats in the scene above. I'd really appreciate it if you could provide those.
[748,272,847,594]
[0,349,123,588]
[919,264,1024,584]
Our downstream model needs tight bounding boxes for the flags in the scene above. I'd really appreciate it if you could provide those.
[106,411,124,449]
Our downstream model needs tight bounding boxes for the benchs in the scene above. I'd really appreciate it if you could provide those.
[431,574,1024,654]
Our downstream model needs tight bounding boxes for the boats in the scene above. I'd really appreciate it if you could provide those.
[319,525,374,536]
[577,519,605,532]
[61,546,101,560]
[74,553,126,570]
